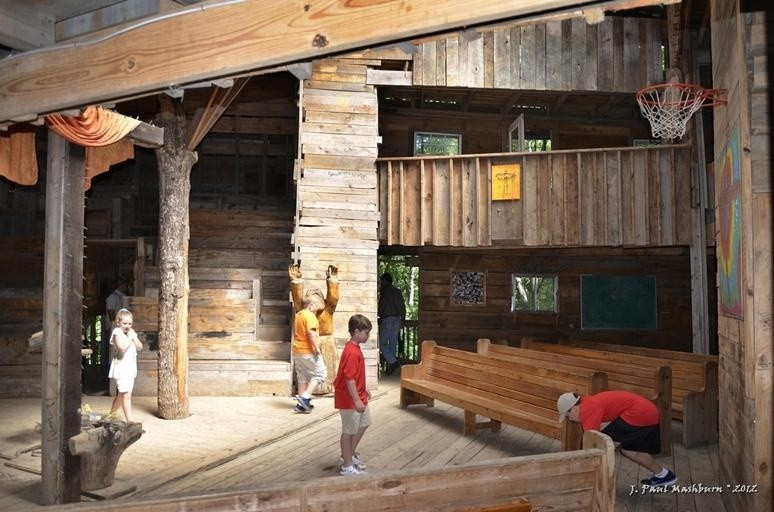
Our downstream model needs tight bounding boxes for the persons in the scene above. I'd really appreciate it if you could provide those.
[556,390,680,487]
[287,259,341,398]
[293,296,327,416]
[99,275,130,397]
[333,314,373,476]
[107,308,147,423]
[377,273,407,375]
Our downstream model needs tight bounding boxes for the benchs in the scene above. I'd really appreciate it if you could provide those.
[396,341,608,452]
[559,339,718,448]
[478,339,672,454]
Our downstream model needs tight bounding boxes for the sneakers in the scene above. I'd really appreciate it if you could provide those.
[294,395,313,413]
[340,455,364,476]
[640,469,677,487]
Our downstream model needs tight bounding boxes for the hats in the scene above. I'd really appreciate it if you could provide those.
[557,392,580,424]
